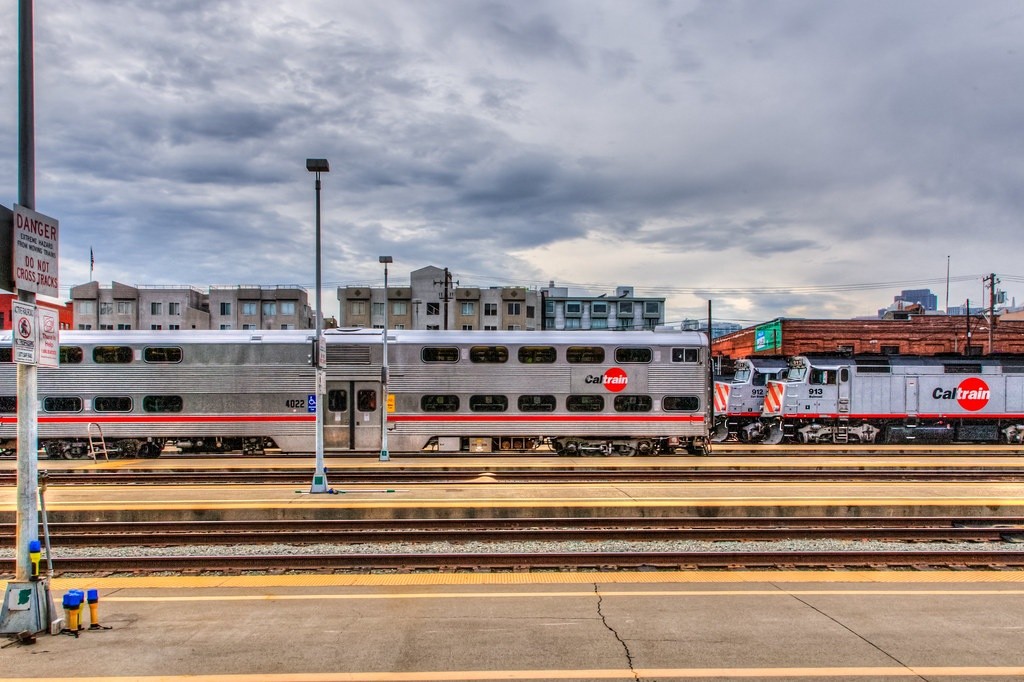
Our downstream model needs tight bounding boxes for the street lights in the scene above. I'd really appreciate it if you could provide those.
[378,255,393,461]
[306,158,329,493]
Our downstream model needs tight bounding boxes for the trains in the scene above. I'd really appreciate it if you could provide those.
[724,353,1024,445]
[0,327,713,457]
